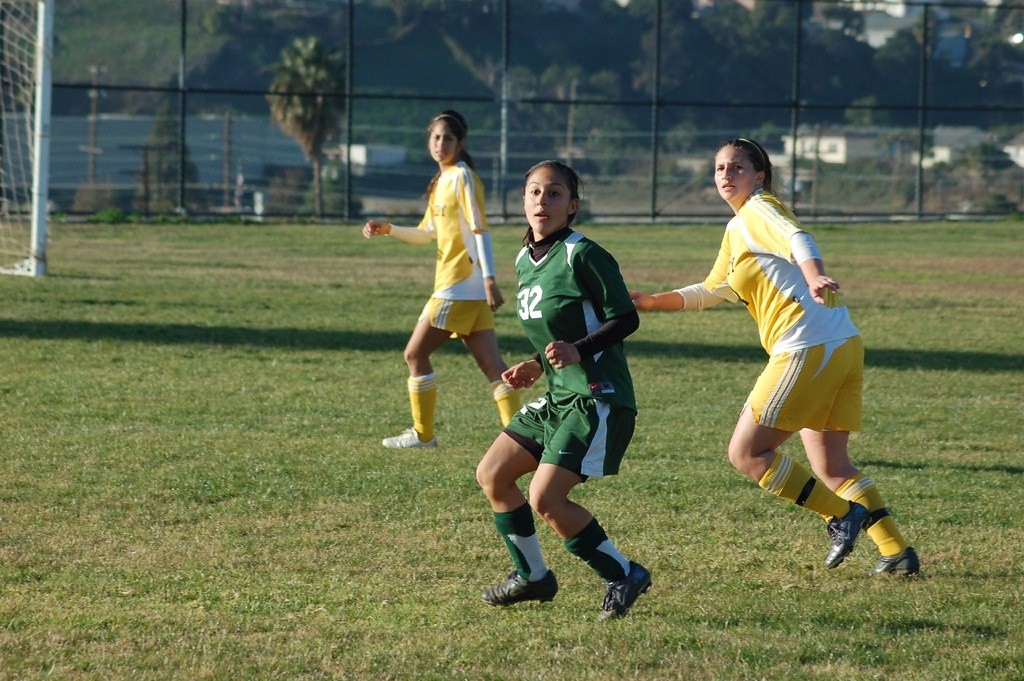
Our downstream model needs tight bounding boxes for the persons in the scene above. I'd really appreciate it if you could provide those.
[361,110,521,448]
[628,137,920,576]
[477,160,652,619]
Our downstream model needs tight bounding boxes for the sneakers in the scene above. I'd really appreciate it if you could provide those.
[868,547,919,576]
[825,500,871,569]
[482,570,558,605]
[382,428,437,448]
[597,561,652,621]
